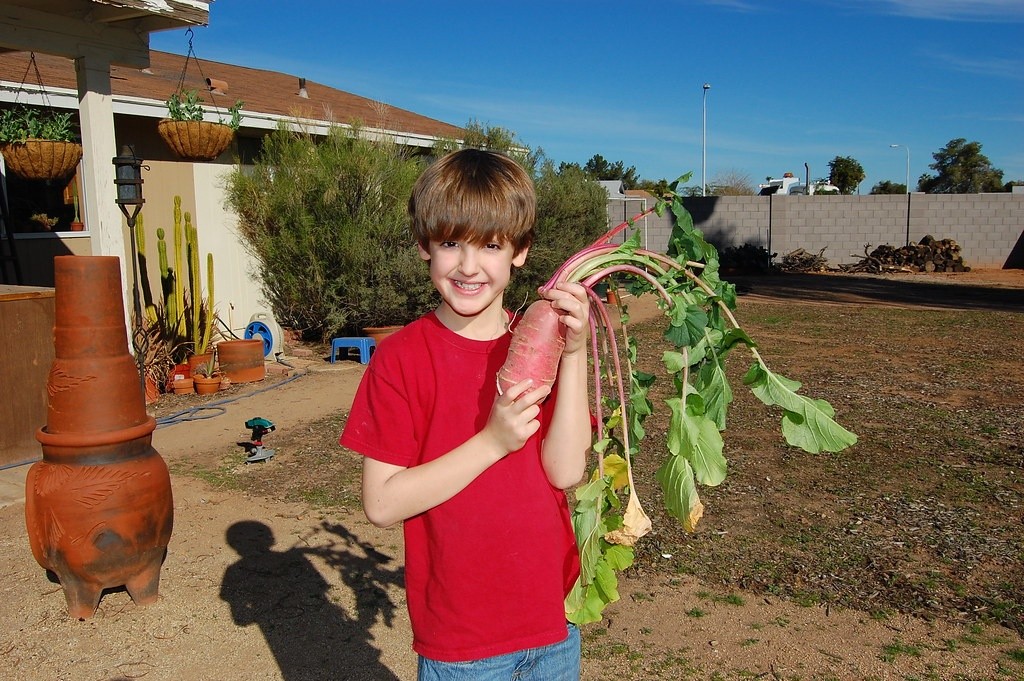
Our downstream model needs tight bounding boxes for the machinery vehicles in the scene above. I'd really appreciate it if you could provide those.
[758,173,841,196]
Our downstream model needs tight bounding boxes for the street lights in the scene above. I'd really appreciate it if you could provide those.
[889,144,910,197]
[702,83,709,197]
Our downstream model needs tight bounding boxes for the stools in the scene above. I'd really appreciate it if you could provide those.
[331,337,376,364]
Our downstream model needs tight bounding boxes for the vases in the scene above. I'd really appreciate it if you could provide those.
[363,325,404,346]
[217,339,266,384]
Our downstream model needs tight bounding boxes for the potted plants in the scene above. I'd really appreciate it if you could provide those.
[0,102,83,186]
[30,214,58,232]
[131,195,221,402]
[604,279,619,304]
[158,88,245,161]
[71,181,83,231]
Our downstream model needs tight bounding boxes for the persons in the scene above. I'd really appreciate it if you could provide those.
[341,148,597,681]
